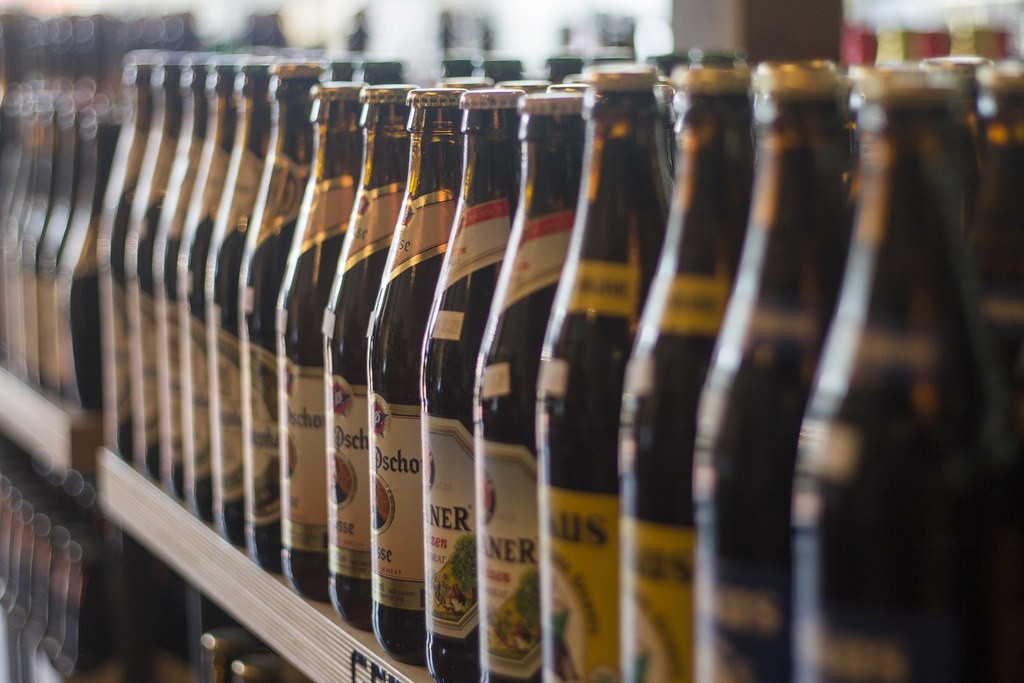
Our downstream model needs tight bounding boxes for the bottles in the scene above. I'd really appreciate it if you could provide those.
[0,8,1022,683]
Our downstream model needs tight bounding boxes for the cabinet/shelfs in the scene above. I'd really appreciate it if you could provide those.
[1,365,433,683]
